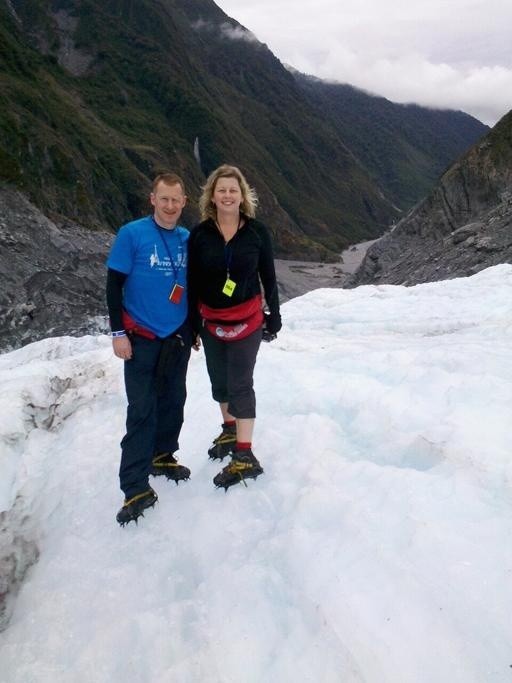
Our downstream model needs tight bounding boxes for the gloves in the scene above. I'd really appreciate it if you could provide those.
[266,312,282,334]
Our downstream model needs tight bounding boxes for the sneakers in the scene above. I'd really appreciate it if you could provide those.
[207,425,237,459]
[214,448,262,489]
[150,452,190,482]
[116,488,157,527]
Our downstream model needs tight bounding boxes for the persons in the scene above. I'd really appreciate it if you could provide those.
[106,173,192,522]
[187,162,284,487]
